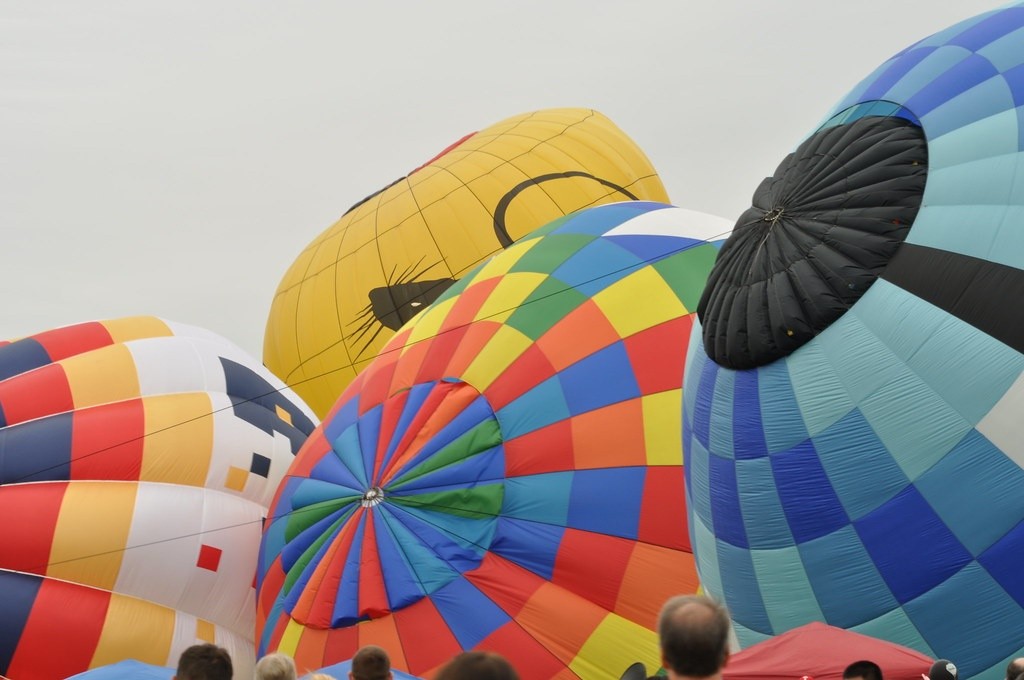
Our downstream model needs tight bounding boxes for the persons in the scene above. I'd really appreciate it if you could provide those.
[843,660,883,680]
[254,653,296,680]
[922,659,958,680]
[348,646,393,680]
[1005,658,1024,680]
[435,651,520,680]
[172,644,233,680]
[656,595,732,680]
